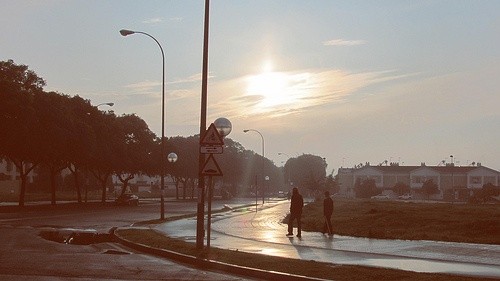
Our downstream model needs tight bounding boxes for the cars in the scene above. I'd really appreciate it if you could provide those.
[116,194,139,206]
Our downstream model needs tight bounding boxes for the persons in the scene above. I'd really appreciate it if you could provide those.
[322,191,334,237]
[285,188,304,237]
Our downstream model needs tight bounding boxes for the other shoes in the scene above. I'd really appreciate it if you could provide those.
[286,233,293,236]
[296,234,302,238]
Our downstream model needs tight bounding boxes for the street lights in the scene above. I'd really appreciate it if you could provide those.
[83,101,114,204]
[449,155,454,192]
[279,152,291,178]
[119,29,166,222]
[242,128,265,204]
